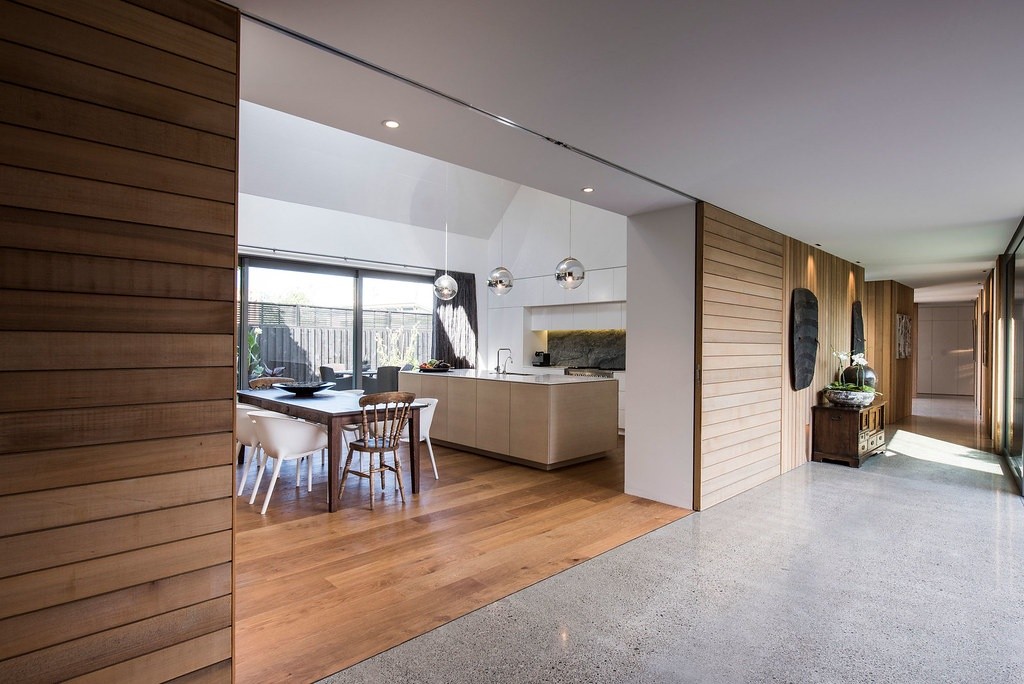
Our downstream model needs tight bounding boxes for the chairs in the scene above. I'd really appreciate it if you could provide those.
[248,377,295,389]
[236,401,301,497]
[316,366,401,393]
[338,392,416,511]
[373,398,440,491]
[248,411,332,515]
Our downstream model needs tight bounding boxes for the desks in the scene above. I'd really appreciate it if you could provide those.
[237,388,430,512]
[333,369,378,378]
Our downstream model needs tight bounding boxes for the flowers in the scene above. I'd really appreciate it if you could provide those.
[247,327,285,379]
[828,343,876,393]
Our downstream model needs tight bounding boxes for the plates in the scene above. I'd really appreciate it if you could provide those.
[416,367,455,372]
[272,381,336,398]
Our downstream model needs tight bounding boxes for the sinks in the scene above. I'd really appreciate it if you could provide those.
[490,373,534,376]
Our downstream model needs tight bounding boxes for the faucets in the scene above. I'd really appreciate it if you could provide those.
[503,356,513,374]
[494,348,511,374]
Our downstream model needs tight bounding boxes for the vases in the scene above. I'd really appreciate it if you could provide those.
[820,387,875,407]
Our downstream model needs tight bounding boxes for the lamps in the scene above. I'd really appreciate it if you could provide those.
[486,214,514,296]
[554,199,585,290]
[432,213,458,300]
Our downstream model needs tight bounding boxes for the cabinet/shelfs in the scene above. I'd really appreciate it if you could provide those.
[810,398,889,469]
